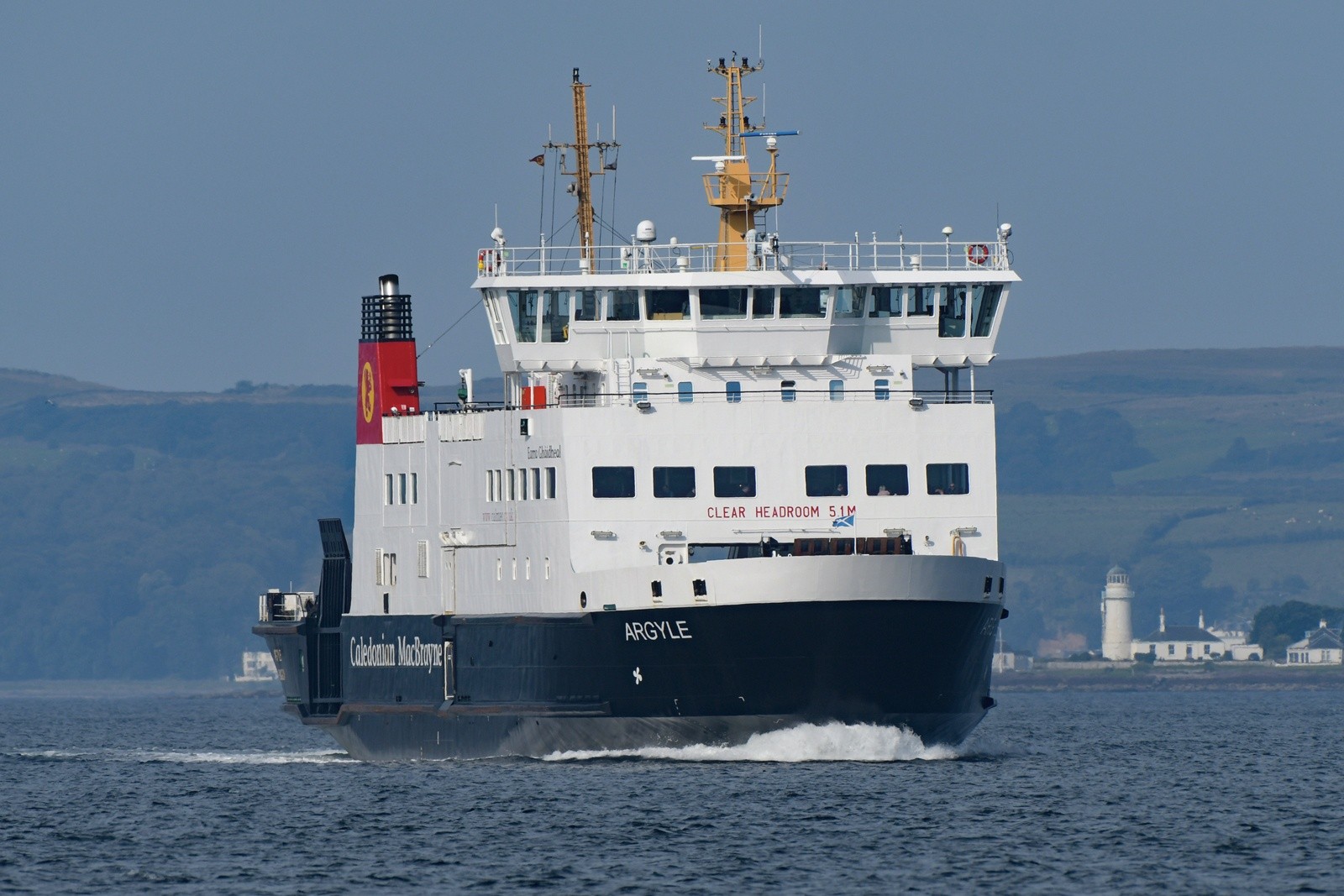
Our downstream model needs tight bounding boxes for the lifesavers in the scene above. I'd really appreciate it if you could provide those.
[476,248,503,271]
[968,244,988,264]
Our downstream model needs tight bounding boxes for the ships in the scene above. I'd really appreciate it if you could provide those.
[249,22,1023,751]
